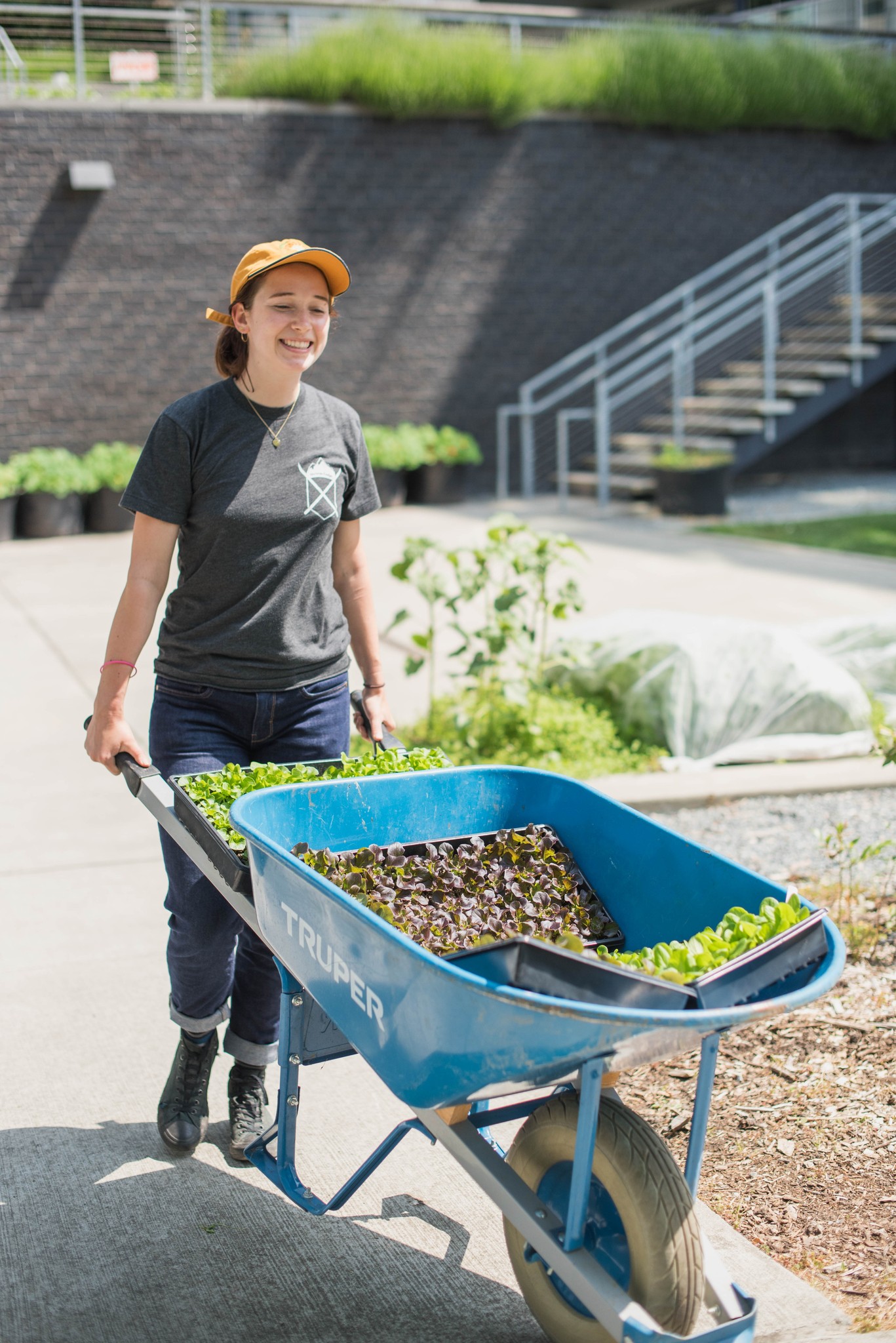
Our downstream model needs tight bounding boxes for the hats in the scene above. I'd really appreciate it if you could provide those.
[205,239,351,330]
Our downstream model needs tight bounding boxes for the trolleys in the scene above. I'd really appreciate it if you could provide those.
[77,686,846,1343]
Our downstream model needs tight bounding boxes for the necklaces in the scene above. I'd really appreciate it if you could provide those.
[233,374,301,448]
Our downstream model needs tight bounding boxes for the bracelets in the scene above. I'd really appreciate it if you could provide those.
[99,661,138,680]
[363,681,385,689]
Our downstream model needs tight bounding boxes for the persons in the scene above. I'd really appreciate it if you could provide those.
[84,235,396,1161]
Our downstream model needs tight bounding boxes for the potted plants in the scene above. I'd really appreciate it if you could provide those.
[0,422,484,540]
[651,439,735,515]
[168,746,830,1011]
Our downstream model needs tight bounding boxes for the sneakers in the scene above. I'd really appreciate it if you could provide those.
[158,1029,219,1151]
[227,1067,269,1161]
